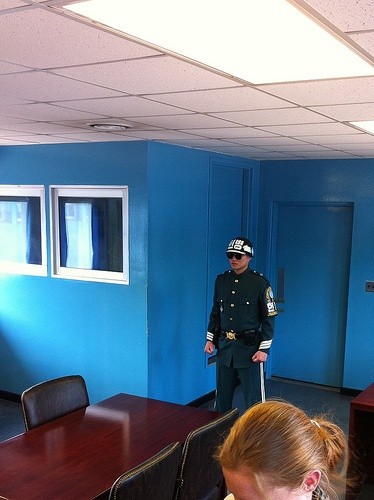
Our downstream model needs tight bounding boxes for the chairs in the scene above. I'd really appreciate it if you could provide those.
[21,374,91,433]
[174,406,240,500]
[108,440,182,500]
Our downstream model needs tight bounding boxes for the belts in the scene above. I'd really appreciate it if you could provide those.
[218,330,246,340]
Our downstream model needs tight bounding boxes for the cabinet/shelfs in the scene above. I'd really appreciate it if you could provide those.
[344,382,374,500]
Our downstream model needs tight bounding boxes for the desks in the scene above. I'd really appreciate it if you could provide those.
[0,393,224,500]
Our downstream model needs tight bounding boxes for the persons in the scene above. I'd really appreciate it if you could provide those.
[213,398,348,500]
[205,236,278,413]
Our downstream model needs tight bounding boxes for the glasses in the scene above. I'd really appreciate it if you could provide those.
[226,253,243,260]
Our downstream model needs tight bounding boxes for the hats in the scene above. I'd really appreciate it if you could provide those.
[227,238,255,257]
